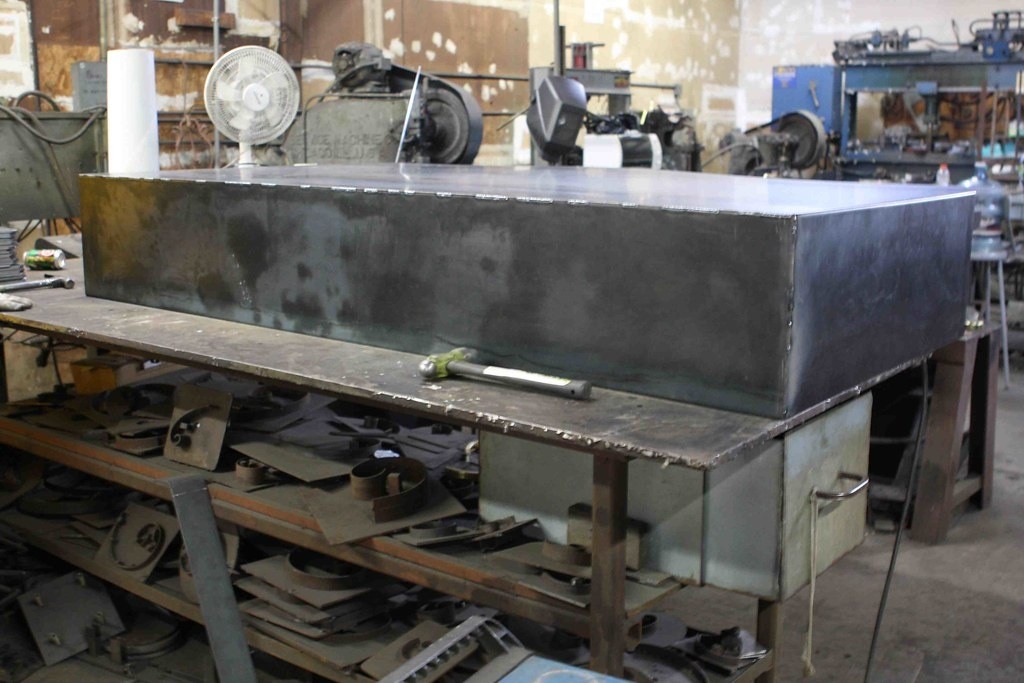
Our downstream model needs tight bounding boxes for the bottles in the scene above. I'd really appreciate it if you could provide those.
[937,165,952,189]
[960,160,1005,258]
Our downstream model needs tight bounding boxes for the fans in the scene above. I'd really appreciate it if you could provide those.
[203,45,300,165]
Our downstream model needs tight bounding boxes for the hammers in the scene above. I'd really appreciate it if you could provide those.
[0,274,75,292]
[419,346,591,402]
